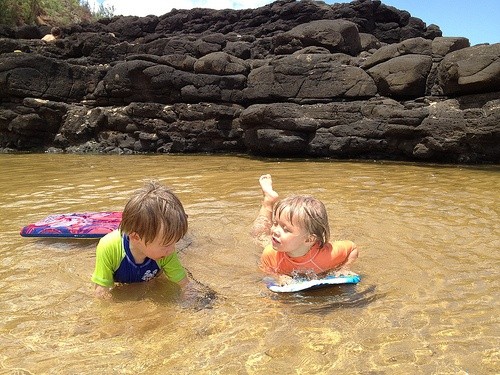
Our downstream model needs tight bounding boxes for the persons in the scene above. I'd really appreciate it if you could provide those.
[250,174,359,286]
[92,180,189,296]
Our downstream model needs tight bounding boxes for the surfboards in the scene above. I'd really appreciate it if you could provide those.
[263,271,361,293]
